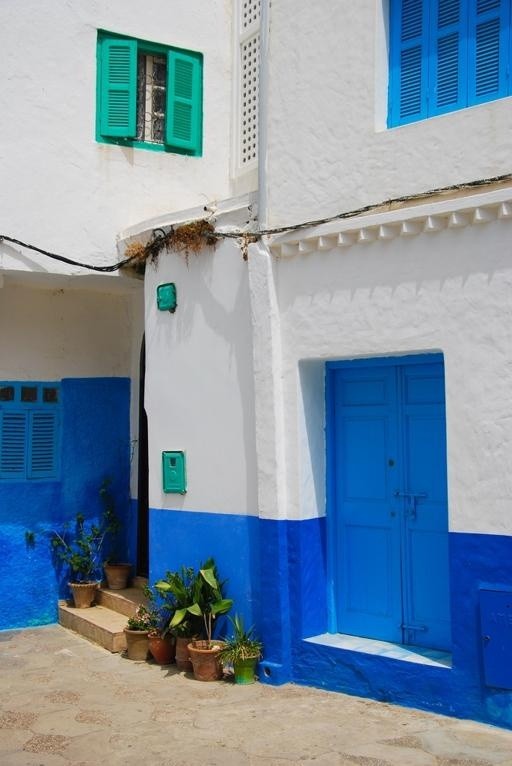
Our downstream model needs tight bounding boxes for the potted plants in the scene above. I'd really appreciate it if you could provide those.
[25,507,113,608]
[99,475,131,590]
[141,555,265,685]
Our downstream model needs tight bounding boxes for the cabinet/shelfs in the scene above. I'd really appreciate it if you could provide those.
[479,581,512,690]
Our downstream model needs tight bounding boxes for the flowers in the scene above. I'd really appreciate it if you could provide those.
[128,602,155,631]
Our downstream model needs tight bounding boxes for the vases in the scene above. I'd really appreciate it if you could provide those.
[124,626,156,661]
